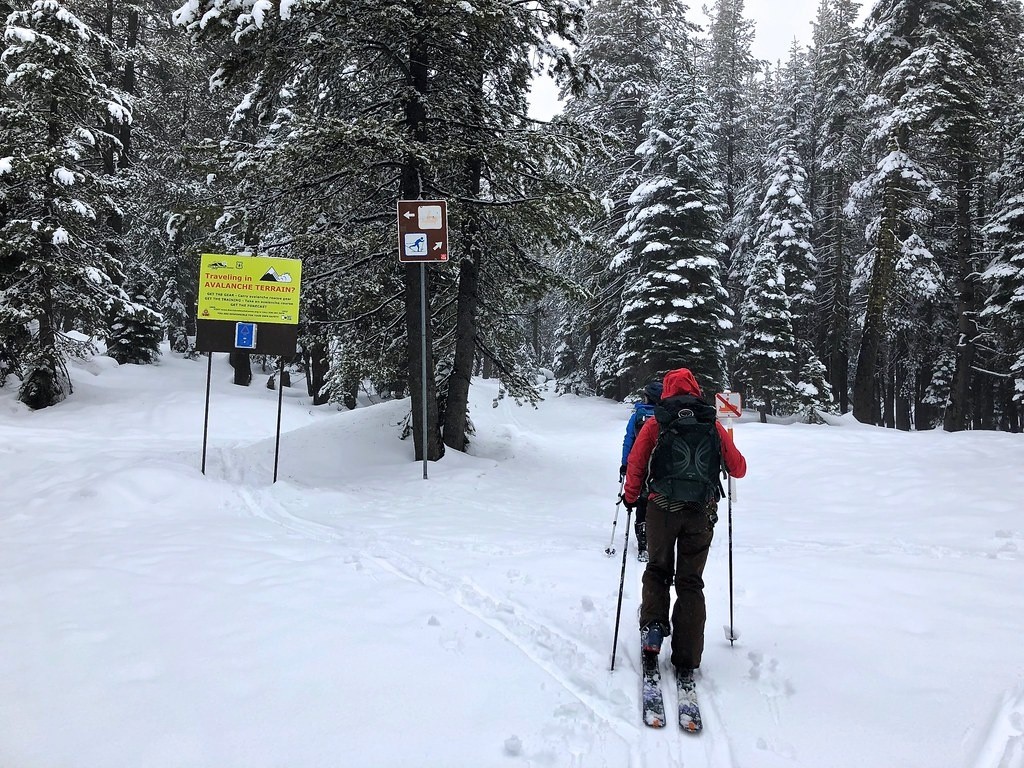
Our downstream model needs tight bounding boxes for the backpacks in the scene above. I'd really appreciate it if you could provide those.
[635,407,655,435]
[644,397,722,513]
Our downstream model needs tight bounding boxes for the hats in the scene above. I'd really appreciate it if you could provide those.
[645,382,664,403]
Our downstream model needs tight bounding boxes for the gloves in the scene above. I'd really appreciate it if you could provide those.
[620,465,627,474]
[622,494,637,508]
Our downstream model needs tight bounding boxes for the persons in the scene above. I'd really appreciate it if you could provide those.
[621,381,664,563]
[624,368,747,682]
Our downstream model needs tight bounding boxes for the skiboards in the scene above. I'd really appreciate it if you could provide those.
[634,521,650,562]
[637,603,704,734]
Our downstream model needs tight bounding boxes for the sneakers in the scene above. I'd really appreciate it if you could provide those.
[640,622,664,654]
[670,655,700,673]
